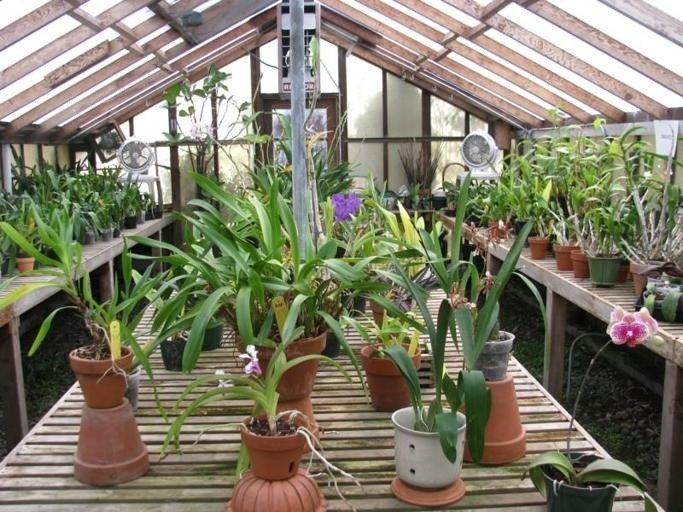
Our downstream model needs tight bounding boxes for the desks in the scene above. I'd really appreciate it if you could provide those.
[0,219,181,445]
[430,210,682,511]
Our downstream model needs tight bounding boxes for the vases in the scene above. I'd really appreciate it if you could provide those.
[539,452,618,510]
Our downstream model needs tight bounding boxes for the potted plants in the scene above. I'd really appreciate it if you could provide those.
[71,171,530,510]
[0,166,165,275]
[516,305,664,511]
[449,114,683,360]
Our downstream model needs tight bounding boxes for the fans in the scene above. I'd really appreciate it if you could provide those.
[111,135,163,208]
